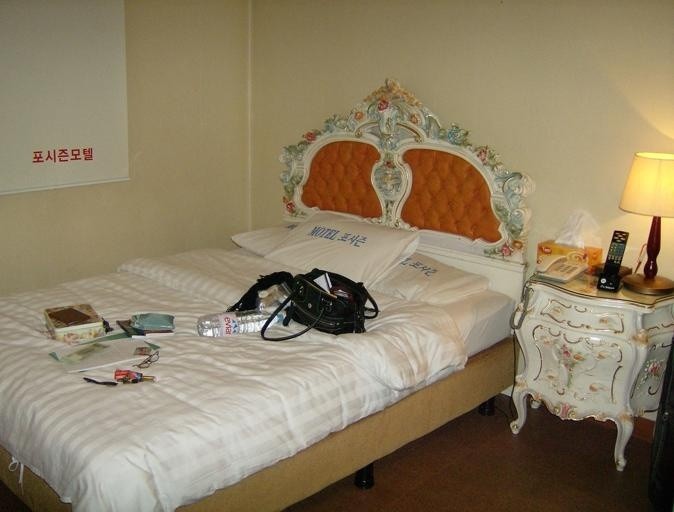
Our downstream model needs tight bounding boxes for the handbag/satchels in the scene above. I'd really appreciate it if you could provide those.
[290,267,364,333]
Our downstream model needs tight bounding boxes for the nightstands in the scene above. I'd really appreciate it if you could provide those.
[504,268,674,472]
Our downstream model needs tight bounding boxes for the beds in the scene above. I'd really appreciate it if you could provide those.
[0,78,531,511]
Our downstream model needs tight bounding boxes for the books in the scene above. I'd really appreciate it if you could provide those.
[45,313,175,373]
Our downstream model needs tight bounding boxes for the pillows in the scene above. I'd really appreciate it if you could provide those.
[228,208,491,308]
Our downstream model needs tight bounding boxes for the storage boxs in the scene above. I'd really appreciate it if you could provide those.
[41,303,102,340]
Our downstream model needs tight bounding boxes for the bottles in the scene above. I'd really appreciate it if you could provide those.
[194,308,285,340]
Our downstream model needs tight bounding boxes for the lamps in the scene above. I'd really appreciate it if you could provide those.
[619,150,674,298]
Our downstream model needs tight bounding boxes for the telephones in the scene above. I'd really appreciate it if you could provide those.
[534,254,590,282]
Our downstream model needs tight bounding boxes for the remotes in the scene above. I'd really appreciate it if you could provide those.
[603,229,630,277]
